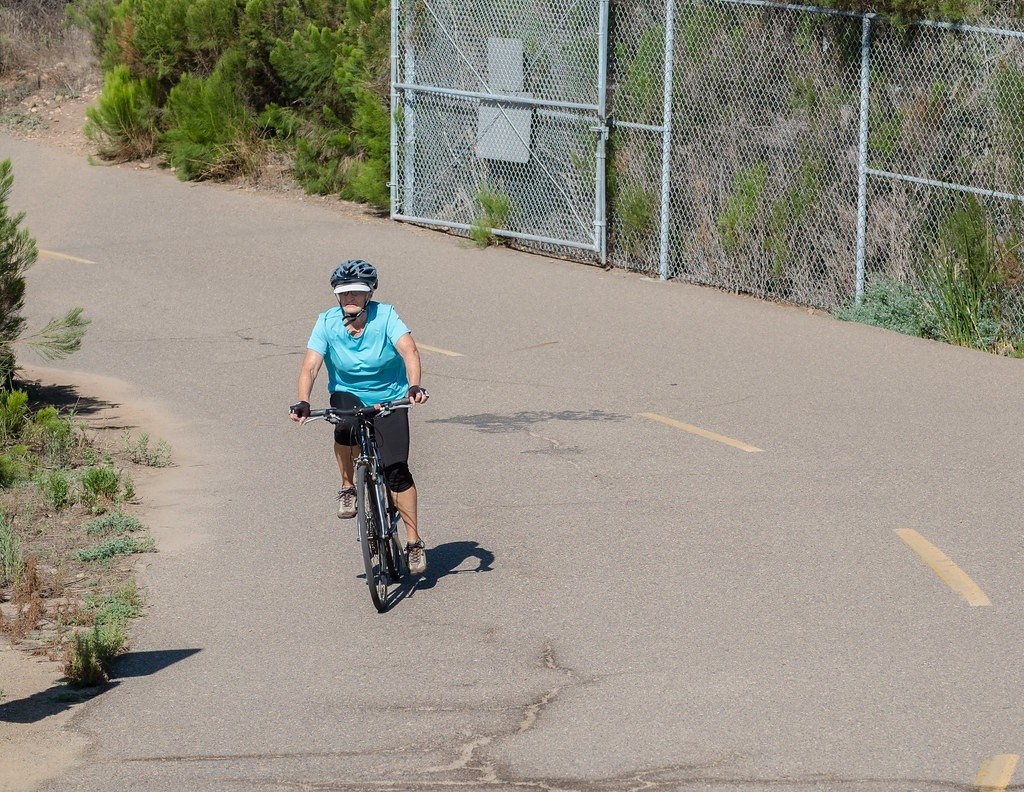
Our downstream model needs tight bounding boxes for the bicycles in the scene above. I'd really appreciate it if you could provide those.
[287,392,430,613]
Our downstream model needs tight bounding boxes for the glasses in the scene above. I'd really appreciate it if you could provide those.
[339,291,367,296]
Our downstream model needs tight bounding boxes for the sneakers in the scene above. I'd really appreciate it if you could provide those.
[405,537,427,575]
[335,483,358,519]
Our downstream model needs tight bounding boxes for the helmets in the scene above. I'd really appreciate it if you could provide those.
[329,260,379,292]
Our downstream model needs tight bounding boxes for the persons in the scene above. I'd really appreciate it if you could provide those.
[289,259,431,576]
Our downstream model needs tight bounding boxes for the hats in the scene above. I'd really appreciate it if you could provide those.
[333,282,371,293]
